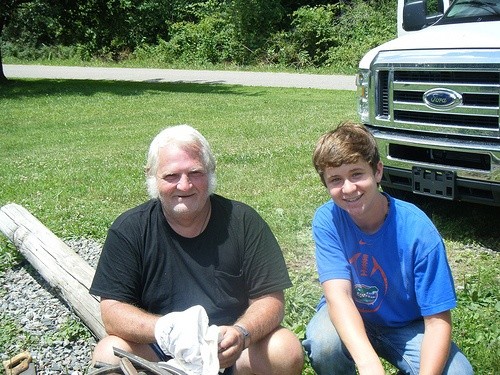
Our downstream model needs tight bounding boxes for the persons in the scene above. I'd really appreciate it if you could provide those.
[87,125,306,375]
[300,118,475,375]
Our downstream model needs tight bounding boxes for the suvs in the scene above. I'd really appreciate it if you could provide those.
[357,0,500,213]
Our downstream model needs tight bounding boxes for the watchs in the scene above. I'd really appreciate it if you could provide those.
[234,324,250,350]
